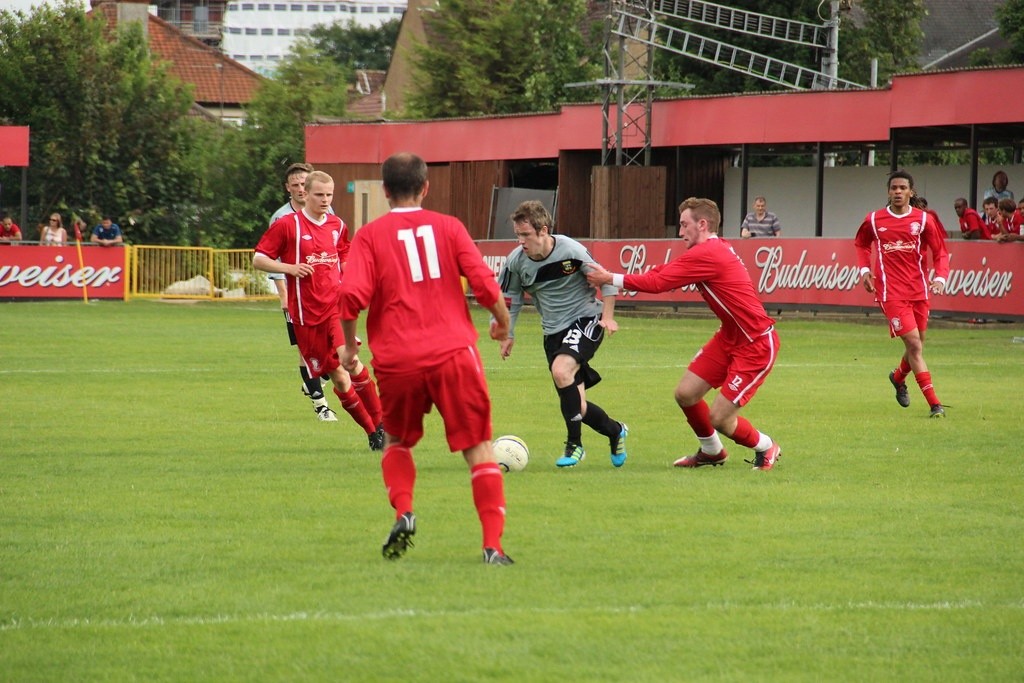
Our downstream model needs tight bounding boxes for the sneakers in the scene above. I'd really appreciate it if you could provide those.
[930,405,945,418]
[315,405,338,421]
[744,439,782,471]
[368,426,385,450]
[609,421,628,467]
[302,384,314,403]
[555,441,585,467]
[484,548,514,565]
[673,448,728,468]
[381,512,416,561]
[889,370,910,407]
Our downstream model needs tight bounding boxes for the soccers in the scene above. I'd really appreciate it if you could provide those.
[491,435,529,472]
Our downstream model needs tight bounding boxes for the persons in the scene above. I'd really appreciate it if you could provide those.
[0,217,23,246]
[336,152,516,565]
[741,198,782,238]
[268,162,338,422]
[954,172,1024,324]
[586,198,782,471]
[854,172,952,419]
[489,200,629,468]
[252,171,386,451]
[39,212,68,247]
[90,216,123,246]
[910,197,949,319]
